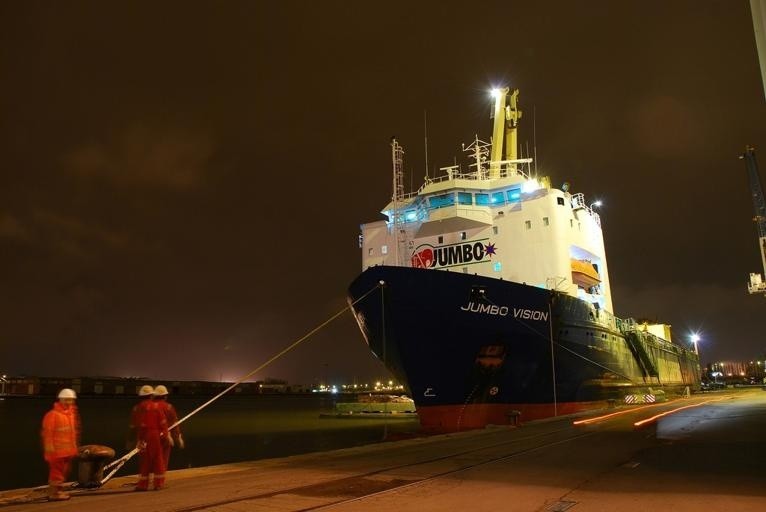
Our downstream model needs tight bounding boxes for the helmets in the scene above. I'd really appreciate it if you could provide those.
[58,389,77,399]
[139,385,154,396]
[155,385,169,396]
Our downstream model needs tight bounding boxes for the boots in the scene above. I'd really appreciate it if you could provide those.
[46,486,70,501]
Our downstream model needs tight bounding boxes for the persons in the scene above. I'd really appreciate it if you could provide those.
[152,386,183,475]
[127,385,174,491]
[43,389,79,500]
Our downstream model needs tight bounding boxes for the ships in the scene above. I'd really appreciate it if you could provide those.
[347,84,706,434]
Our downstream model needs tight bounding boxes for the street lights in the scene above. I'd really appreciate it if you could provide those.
[712,361,763,377]
